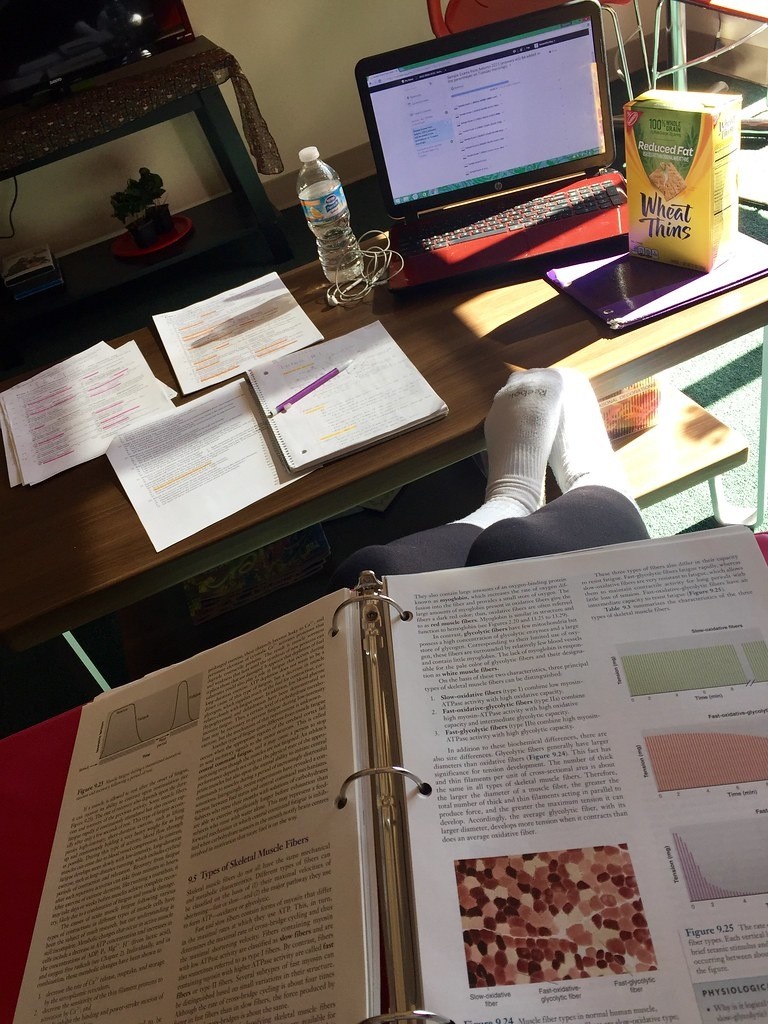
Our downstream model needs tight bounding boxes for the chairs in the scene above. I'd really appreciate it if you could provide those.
[427,0,652,130]
[653,0,767,118]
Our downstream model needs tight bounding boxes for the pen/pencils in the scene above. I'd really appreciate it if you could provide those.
[269,360,354,416]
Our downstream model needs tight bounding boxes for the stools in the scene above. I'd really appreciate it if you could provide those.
[467,377,750,537]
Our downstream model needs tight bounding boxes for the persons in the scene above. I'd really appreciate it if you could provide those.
[325,358,654,587]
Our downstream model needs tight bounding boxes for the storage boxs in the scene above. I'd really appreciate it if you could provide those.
[621,86,747,275]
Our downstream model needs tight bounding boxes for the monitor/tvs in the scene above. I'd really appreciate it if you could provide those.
[0,0,195,110]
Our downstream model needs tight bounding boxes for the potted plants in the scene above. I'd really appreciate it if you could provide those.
[109,167,193,261]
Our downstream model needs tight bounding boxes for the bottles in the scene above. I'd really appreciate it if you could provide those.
[296,146,367,284]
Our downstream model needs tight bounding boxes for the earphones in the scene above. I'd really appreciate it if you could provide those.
[326,292,337,308]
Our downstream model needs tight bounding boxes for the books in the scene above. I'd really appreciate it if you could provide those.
[0,521,767,1024]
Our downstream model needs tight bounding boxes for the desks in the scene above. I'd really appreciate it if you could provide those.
[0,36,294,267]
[0,157,768,700]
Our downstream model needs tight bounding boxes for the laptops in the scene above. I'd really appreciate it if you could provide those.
[352,0,627,293]
